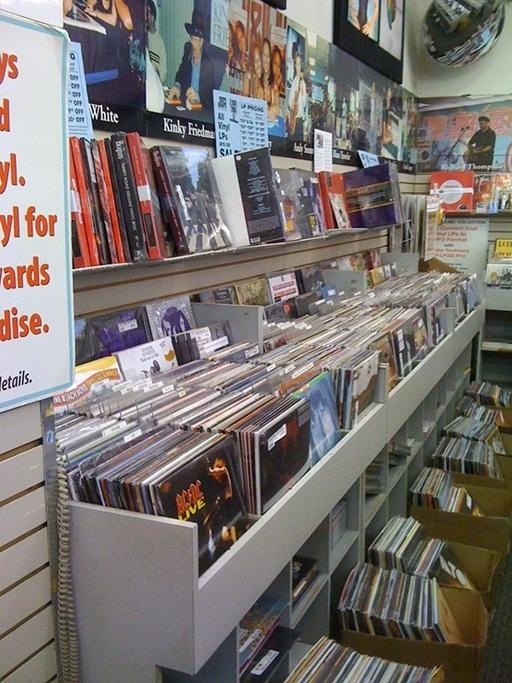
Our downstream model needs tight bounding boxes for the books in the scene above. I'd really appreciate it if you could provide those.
[236,379,512,682]
[60,272,512,614]
[66,131,511,289]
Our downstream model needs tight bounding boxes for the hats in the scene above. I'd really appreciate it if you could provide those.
[185,7,209,37]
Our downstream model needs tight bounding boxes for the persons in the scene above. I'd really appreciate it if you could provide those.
[204,454,238,545]
[466,115,497,169]
[162,312,188,336]
[308,382,340,460]
[83,0,418,166]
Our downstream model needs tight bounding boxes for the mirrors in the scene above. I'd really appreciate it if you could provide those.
[421,0,506,67]
